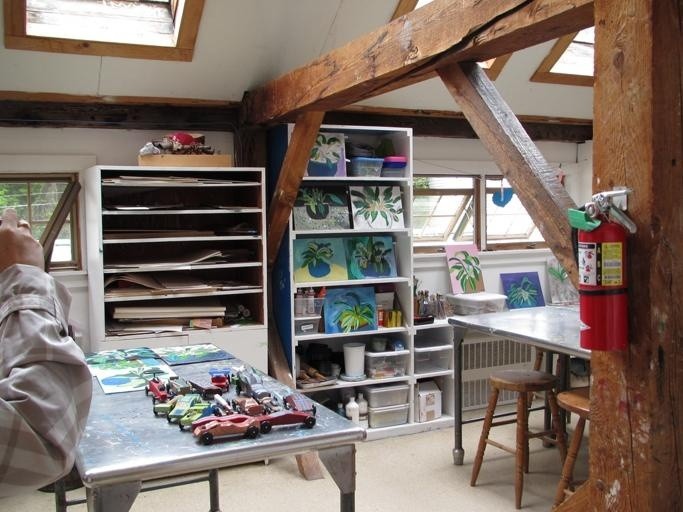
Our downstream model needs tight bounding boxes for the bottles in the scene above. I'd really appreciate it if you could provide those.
[295,286,325,314]
[418,289,444,320]
[338,393,368,429]
[377,304,384,326]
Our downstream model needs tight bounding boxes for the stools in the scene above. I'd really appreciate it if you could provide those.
[553,386,592,511]
[471,368,560,507]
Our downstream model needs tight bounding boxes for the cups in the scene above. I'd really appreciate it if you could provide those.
[342,342,365,377]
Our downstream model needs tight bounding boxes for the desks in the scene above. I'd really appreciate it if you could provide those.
[447,304,591,467]
[67,342,365,512]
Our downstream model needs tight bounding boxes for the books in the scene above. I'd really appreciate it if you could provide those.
[100,168,262,338]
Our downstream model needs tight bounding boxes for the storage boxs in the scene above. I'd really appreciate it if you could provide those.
[365,348,409,376]
[413,336,454,375]
[364,409,409,427]
[135,152,232,169]
[293,297,324,316]
[356,386,411,405]
[294,315,322,335]
[349,156,384,178]
[447,291,507,316]
[415,379,441,424]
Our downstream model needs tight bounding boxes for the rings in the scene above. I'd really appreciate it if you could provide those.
[17,221,30,229]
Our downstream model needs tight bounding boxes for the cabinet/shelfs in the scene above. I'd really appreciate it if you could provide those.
[414,320,454,432]
[263,123,414,440]
[83,165,267,378]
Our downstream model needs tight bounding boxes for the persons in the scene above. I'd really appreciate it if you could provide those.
[0,205,93,502]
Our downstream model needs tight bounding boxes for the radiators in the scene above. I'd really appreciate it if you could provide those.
[459,336,539,413]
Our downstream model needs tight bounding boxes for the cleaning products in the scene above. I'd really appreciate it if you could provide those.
[344,397,361,427]
[335,403,346,417]
[355,392,369,430]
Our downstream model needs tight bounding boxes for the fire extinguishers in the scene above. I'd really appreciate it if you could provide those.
[568,190,637,353]
